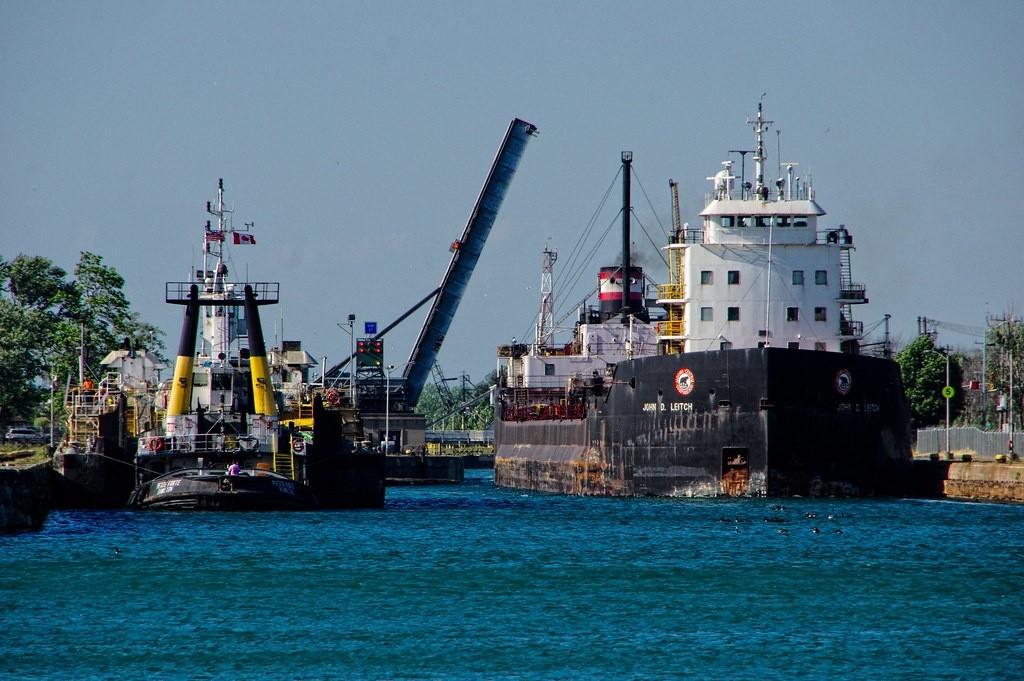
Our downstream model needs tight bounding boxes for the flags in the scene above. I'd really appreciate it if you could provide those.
[233,230,256,244]
[207,230,225,241]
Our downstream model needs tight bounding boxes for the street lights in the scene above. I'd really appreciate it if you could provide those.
[924,350,951,451]
[983,319,1016,432]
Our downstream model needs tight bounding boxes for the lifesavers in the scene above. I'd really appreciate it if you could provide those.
[94,390,105,403]
[1008,441,1011,450]
[149,437,162,451]
[292,439,305,452]
[657,323,659,333]
[163,393,167,410]
[328,392,341,406]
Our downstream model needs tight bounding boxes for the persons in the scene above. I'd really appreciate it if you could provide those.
[229,460,241,476]
[83,375,95,391]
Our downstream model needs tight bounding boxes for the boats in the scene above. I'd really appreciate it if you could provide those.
[131,177,390,515]
[492,93,914,500]
[51,323,167,512]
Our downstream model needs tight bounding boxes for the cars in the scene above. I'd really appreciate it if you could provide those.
[5,428,50,442]
